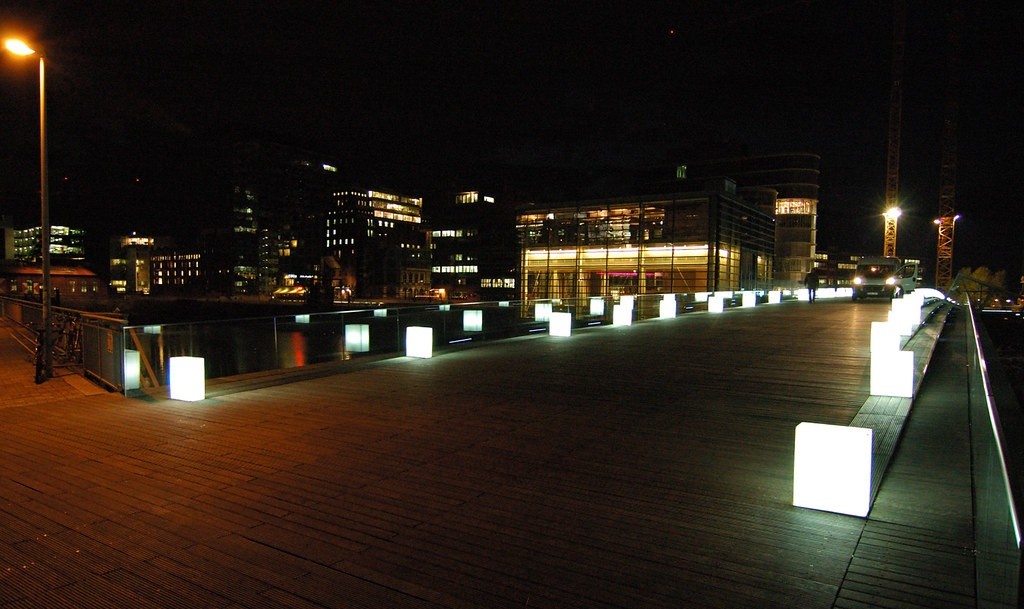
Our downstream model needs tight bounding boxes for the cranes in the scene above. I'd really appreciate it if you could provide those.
[883,3,903,269]
[931,96,952,290]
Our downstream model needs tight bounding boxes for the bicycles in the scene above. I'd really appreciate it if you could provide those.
[52,310,84,373]
[22,321,57,385]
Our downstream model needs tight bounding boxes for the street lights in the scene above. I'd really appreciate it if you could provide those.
[3,37,55,377]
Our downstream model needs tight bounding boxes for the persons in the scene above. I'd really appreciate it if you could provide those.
[805,268,820,304]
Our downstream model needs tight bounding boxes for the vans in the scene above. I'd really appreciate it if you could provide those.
[852,255,917,302]
